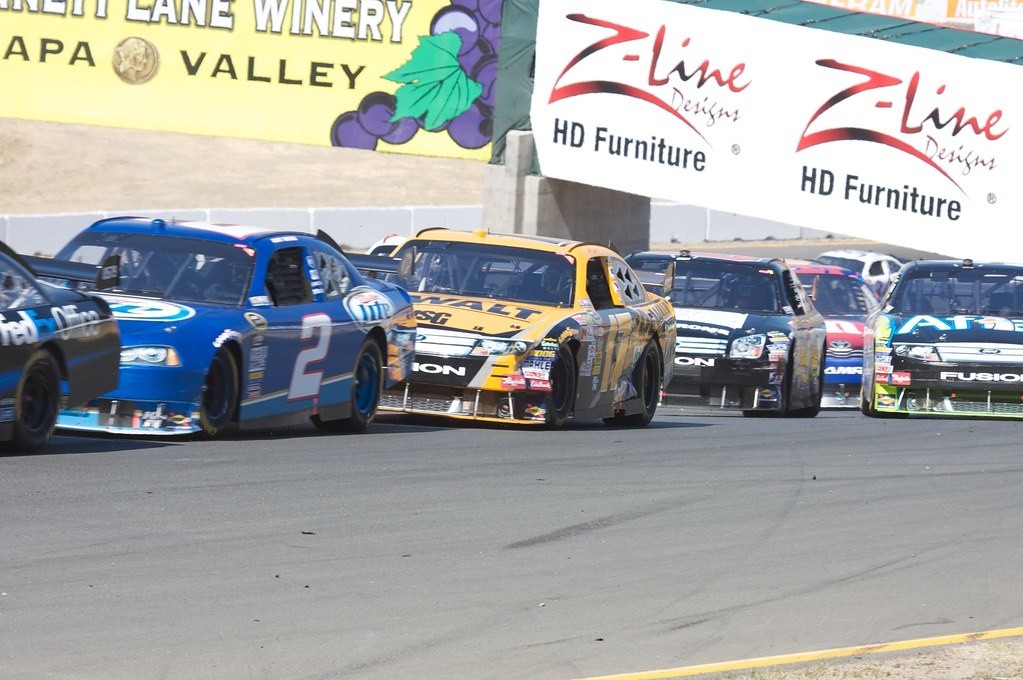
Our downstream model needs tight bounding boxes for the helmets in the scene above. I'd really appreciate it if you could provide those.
[824,279,850,311]
[541,266,571,297]
[230,249,257,289]
[1009,282,1023,314]
[719,265,765,308]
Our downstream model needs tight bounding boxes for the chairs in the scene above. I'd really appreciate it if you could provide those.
[205,259,278,281]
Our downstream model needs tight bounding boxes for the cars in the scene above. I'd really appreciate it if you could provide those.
[783,259,880,408]
[818,250,903,302]
[623,250,827,418]
[859,258,1023,418]
[366,226,680,430]
[367,234,409,278]
[38,215,418,440]
[0,243,123,457]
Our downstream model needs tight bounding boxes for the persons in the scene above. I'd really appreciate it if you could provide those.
[526,262,576,308]
[726,262,774,311]
[199,251,278,306]
